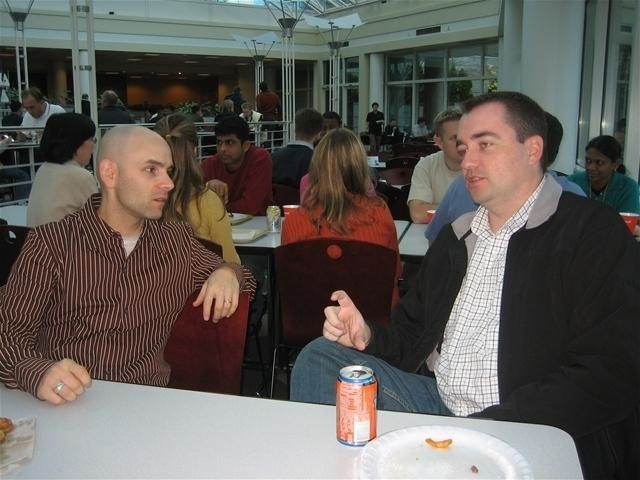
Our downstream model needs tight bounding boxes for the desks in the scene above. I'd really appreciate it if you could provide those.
[6,140,41,200]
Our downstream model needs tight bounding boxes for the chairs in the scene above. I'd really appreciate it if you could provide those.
[361,124,436,198]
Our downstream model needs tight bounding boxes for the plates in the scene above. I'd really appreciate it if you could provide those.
[231,227,268,244]
[360,424,534,480]
[226,212,254,227]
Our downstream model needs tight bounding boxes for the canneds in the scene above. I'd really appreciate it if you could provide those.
[337,366,377,448]
[266,205,281,233]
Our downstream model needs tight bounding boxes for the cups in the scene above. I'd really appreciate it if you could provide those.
[28,130,44,144]
[424,209,436,222]
[282,205,301,218]
[618,211,640,235]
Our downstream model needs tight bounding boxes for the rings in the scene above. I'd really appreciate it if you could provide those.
[224,298,231,303]
[53,382,63,395]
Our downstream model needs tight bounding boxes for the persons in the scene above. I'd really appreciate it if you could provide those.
[2,78,639,275]
[289,90,638,478]
[0,123,258,406]
[279,128,403,312]
